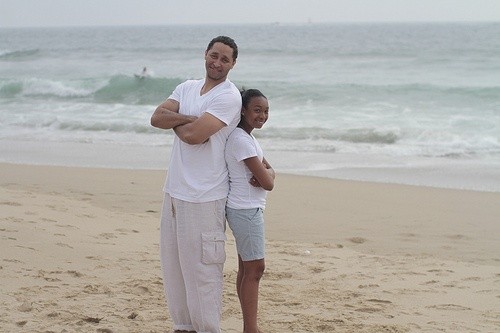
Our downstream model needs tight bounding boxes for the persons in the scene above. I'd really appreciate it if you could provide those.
[150,35,248,333]
[224,84,277,333]
